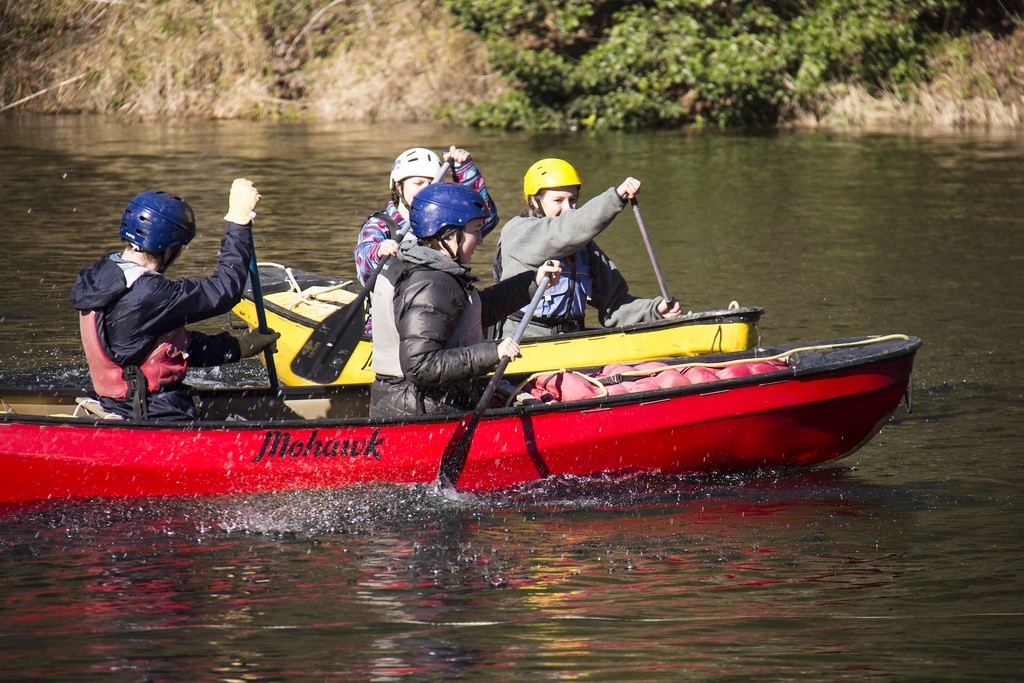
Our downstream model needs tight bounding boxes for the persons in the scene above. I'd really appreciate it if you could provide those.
[354,145,563,419]
[492,156,684,340]
[69,177,281,422]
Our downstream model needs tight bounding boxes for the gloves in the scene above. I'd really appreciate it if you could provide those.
[238,326,280,360]
[223,177,261,226]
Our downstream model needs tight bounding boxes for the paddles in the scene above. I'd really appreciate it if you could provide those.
[623,191,673,313]
[289,151,454,384]
[246,219,280,389]
[437,261,554,495]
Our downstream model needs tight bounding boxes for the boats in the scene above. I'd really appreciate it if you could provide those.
[227,260,768,390]
[0,334,924,509]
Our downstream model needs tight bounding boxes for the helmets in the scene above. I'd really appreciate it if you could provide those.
[389,147,445,190]
[118,190,197,255]
[521,157,582,207]
[409,182,491,238]
